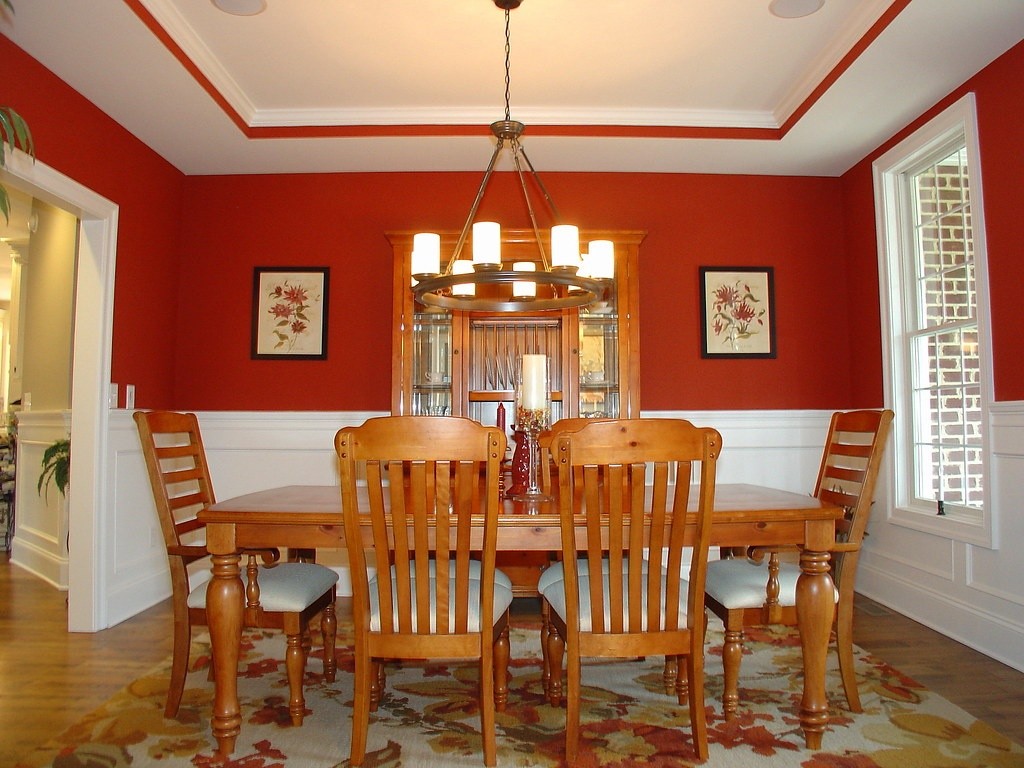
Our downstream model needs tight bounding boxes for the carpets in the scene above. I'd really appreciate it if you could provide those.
[11,598,1024,768]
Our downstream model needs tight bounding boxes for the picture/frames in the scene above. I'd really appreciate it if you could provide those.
[697,263,777,362]
[249,264,331,362]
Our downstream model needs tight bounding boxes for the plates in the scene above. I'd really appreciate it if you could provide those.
[584,379,608,384]
[427,381,447,386]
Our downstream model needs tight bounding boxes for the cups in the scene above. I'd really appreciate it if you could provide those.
[424,371,445,382]
[587,370,605,381]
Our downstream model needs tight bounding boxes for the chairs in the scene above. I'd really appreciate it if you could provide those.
[540,418,725,767]
[694,409,894,752]
[333,415,508,767]
[536,417,673,701]
[131,408,341,729]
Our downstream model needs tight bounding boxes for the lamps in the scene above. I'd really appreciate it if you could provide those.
[407,0,615,313]
